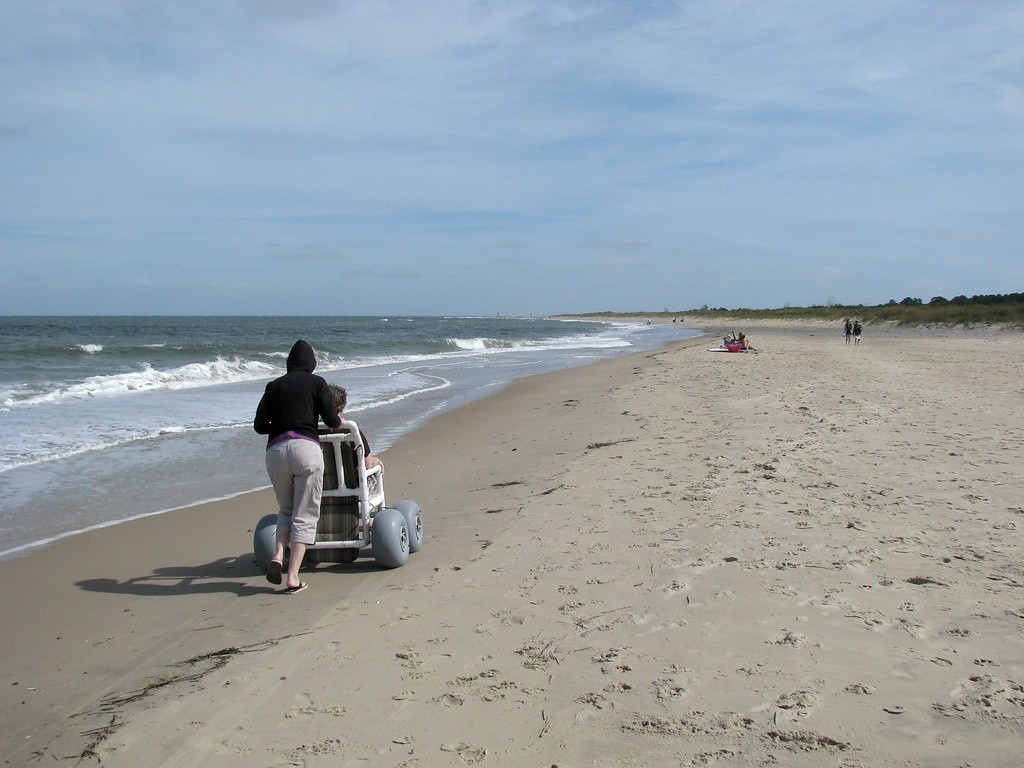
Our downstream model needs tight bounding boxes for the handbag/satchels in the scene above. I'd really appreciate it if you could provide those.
[843,333,846,337]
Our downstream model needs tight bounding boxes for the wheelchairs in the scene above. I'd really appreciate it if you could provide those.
[253,420,423,568]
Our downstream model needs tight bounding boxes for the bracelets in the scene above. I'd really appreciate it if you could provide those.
[376,460,381,465]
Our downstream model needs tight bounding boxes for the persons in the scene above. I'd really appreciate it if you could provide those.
[844,320,862,345]
[253,340,343,594]
[321,384,384,529]
[727,331,751,350]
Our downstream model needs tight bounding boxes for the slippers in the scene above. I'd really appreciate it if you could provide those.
[284,580,309,595]
[266,559,283,586]
